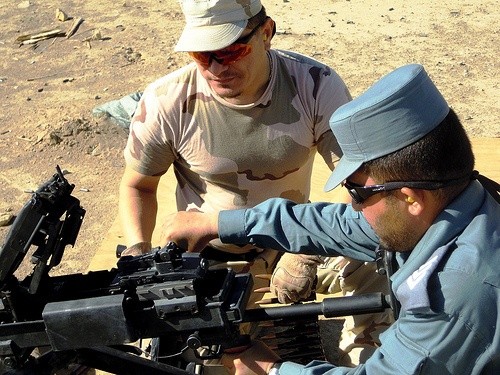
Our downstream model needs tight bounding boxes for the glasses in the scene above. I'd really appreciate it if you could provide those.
[341,181,447,204]
[188,16,276,68]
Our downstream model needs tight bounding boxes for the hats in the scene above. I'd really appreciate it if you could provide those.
[174,0,262,51]
[324,64,450,192]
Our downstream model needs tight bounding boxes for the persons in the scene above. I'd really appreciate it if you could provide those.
[120,0,391,368]
[160,65,500,375]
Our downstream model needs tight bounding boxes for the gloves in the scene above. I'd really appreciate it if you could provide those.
[120,242,152,256]
[270,252,325,304]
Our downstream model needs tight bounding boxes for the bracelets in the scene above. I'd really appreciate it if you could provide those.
[266,357,282,375]
